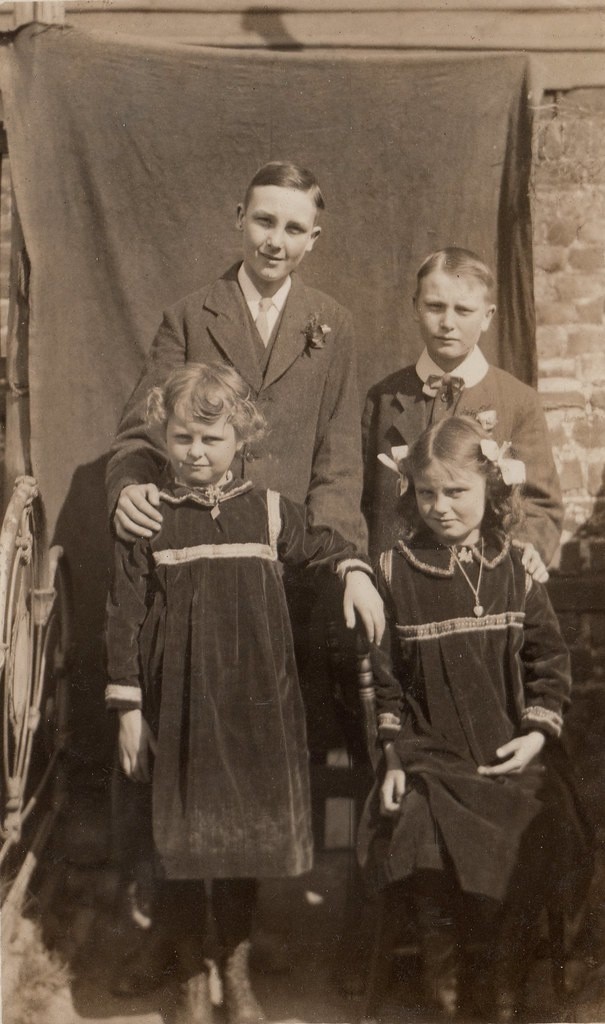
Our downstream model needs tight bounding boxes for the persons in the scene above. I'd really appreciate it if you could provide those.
[354,411,598,1024]
[99,159,370,931]
[103,362,386,1024]
[358,248,564,586]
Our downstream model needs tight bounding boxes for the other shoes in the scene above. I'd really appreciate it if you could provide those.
[335,965,367,995]
[216,928,268,973]
[109,925,177,998]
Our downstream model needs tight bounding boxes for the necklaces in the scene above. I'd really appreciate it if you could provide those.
[176,480,225,520]
[444,535,503,617]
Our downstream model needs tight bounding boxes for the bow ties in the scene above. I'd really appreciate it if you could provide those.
[426,374,466,392]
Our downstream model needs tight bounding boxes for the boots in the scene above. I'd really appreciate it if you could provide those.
[172,941,212,1024]
[216,942,266,1024]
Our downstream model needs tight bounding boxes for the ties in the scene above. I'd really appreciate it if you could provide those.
[256,297,273,349]
